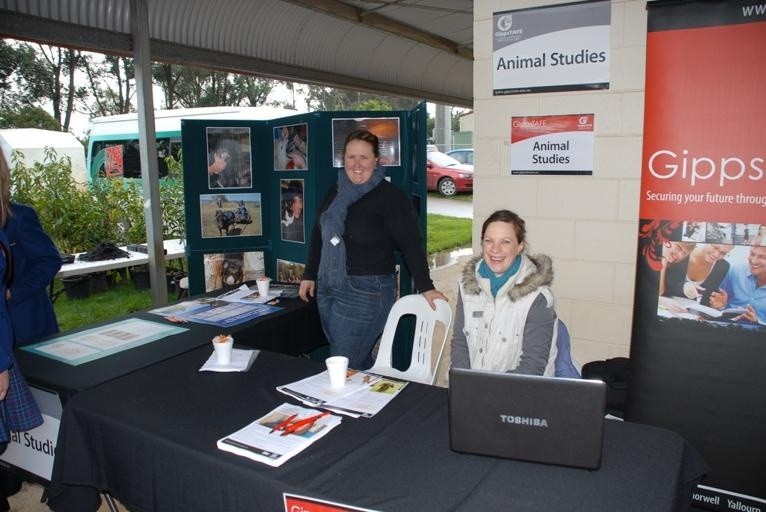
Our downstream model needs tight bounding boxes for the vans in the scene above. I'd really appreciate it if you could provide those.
[88,107,303,200]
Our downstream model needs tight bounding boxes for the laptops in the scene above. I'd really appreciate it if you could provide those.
[447,366,607,471]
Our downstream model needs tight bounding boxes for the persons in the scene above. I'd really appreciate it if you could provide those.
[299,129,448,371]
[666,244,734,308]
[0,144,63,347]
[448,210,559,377]
[274,127,306,170]
[283,181,303,243]
[659,241,696,296]
[749,223,765,246]
[708,247,766,325]
[0,198,44,512]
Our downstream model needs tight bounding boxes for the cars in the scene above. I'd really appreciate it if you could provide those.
[427,152,473,196]
[444,149,472,167]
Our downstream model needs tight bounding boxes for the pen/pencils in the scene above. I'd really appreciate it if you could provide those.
[280,408,333,436]
[268,412,299,435]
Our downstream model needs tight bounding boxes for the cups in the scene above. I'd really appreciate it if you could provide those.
[326,356,349,390]
[212,335,233,367]
[256,277,271,297]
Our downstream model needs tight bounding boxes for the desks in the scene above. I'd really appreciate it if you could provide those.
[50,341,711,512]
[48,238,187,303]
[15,279,333,503]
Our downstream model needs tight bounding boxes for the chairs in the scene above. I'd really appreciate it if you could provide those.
[361,294,452,385]
